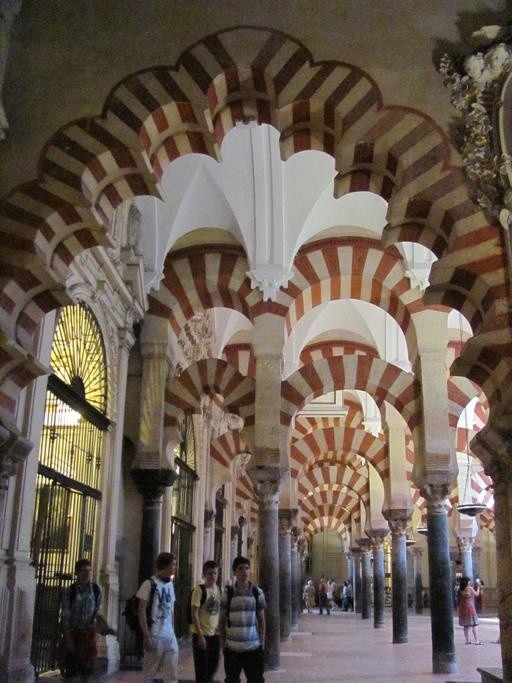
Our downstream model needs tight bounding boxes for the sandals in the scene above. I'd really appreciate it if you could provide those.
[464,641,482,644]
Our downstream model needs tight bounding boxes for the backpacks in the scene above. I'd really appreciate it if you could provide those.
[186,583,207,624]
[123,577,155,632]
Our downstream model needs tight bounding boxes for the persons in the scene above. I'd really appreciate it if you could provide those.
[189,560,223,683]
[218,556,266,683]
[135,552,179,683]
[457,578,481,644]
[303,577,353,615]
[56,560,102,683]
